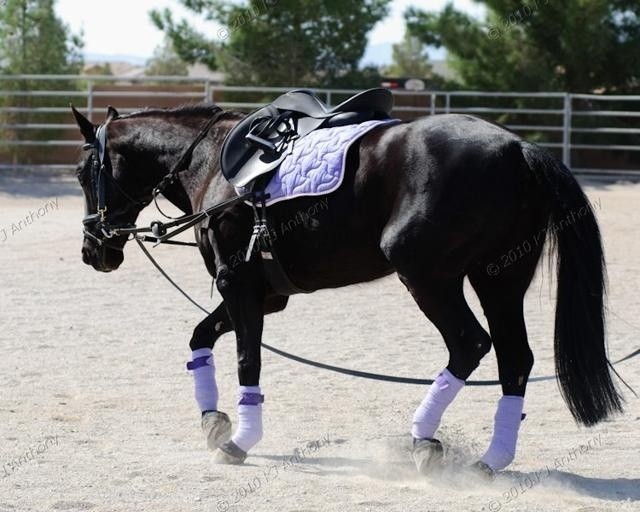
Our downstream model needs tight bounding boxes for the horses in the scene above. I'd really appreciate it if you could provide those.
[67,100,639,490]
